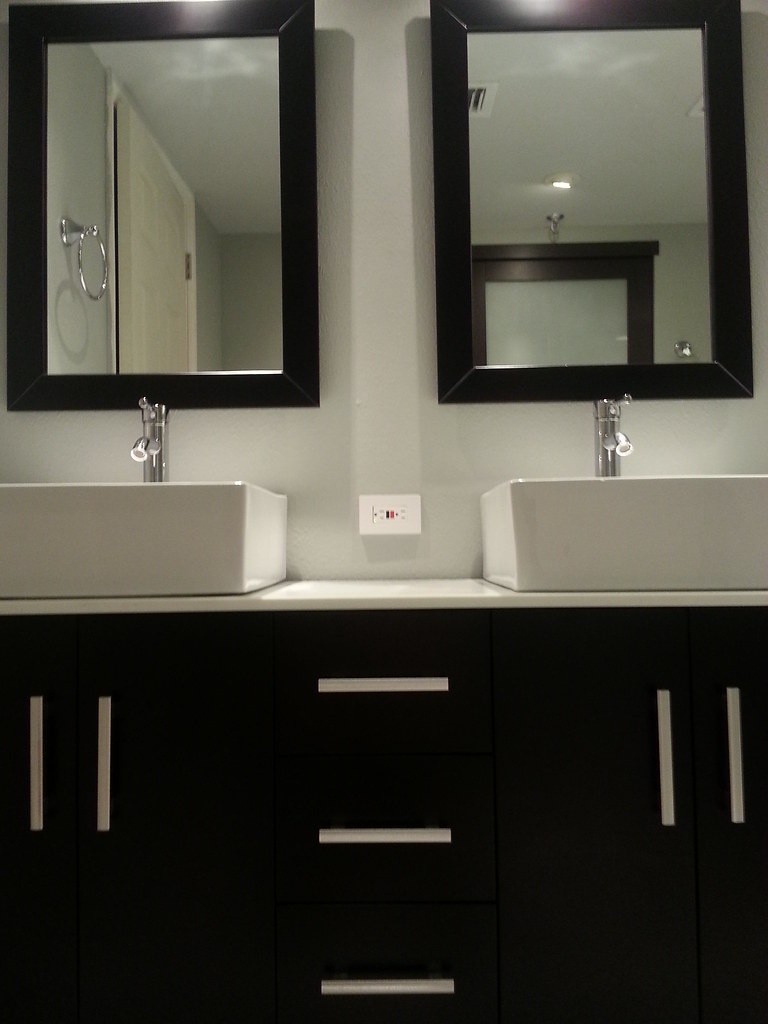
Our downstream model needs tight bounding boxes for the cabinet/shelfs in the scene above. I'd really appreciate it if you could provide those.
[0,604,768,1024]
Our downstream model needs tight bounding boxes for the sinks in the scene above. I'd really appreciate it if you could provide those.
[0,480,288,599]
[479,473,767,592]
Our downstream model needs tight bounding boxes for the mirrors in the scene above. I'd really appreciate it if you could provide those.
[7,0,319,409]
[430,0,753,404]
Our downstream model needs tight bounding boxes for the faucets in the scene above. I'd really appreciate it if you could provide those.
[592,392,634,476]
[129,397,169,482]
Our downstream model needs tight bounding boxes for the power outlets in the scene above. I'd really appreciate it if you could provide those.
[358,494,421,536]
